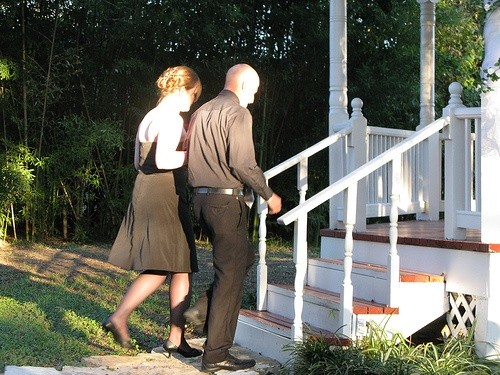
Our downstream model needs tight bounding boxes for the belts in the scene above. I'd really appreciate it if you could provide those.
[192,188,244,196]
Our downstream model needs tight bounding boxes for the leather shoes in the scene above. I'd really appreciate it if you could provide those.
[201,353,256,372]
[183,306,207,336]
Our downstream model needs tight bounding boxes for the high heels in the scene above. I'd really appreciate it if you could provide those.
[101,315,134,350]
[163,338,203,359]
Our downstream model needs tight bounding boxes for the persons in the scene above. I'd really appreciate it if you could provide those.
[102,65,203,359]
[182,63,281,372]
